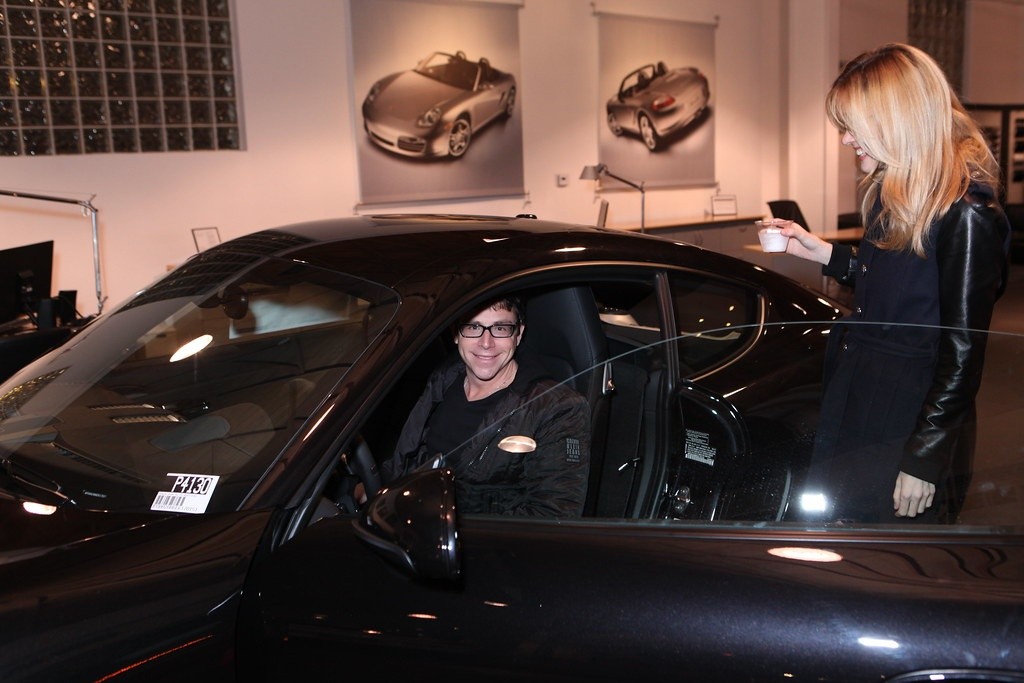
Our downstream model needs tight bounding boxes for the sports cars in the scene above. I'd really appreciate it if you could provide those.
[361,52,516,160]
[605,59,710,155]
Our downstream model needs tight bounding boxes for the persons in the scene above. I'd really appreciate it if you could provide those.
[353,298,591,517]
[756,42,1001,525]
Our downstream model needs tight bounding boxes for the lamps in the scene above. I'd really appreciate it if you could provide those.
[581,164,645,233]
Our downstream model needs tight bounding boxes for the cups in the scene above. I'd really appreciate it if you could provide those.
[755,220,793,252]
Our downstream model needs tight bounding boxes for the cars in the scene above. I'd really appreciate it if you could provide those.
[1,207,1024,683]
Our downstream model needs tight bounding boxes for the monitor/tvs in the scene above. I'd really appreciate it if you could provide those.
[0,241,54,326]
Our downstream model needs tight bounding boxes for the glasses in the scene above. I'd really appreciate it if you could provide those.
[459,318,523,338]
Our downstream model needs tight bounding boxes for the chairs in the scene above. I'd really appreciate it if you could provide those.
[766,200,810,231]
[513,284,610,414]
[362,295,431,343]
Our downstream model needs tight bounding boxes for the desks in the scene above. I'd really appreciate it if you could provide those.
[745,228,864,270]
[621,213,764,234]
